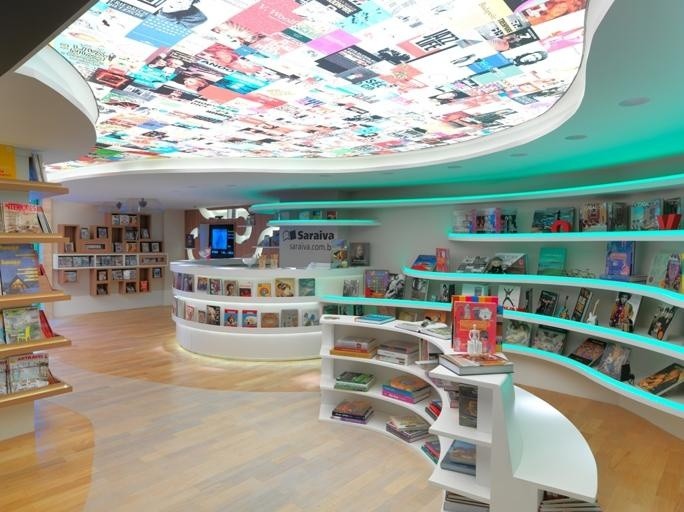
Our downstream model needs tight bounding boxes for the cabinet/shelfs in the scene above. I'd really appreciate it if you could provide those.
[52,212,168,297]
[318,314,601,512]
[250,177,684,419]
[0,178,72,407]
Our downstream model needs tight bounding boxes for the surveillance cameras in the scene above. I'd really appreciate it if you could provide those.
[139,198,147,207]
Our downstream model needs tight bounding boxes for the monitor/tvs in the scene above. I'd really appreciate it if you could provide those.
[209,224,234,258]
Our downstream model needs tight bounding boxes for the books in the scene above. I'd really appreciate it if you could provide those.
[412,248,527,274]
[440,439,477,475]
[330,338,444,365]
[449,204,517,235]
[333,241,370,268]
[517,197,684,408]
[424,368,477,427]
[443,491,490,512]
[331,400,375,425]
[0,143,60,398]
[336,372,376,391]
[343,269,521,309]
[167,274,329,331]
[385,413,441,464]
[331,303,447,325]
[51,215,164,294]
[439,295,514,375]
[382,375,432,404]
[395,321,452,340]
[537,490,602,512]
